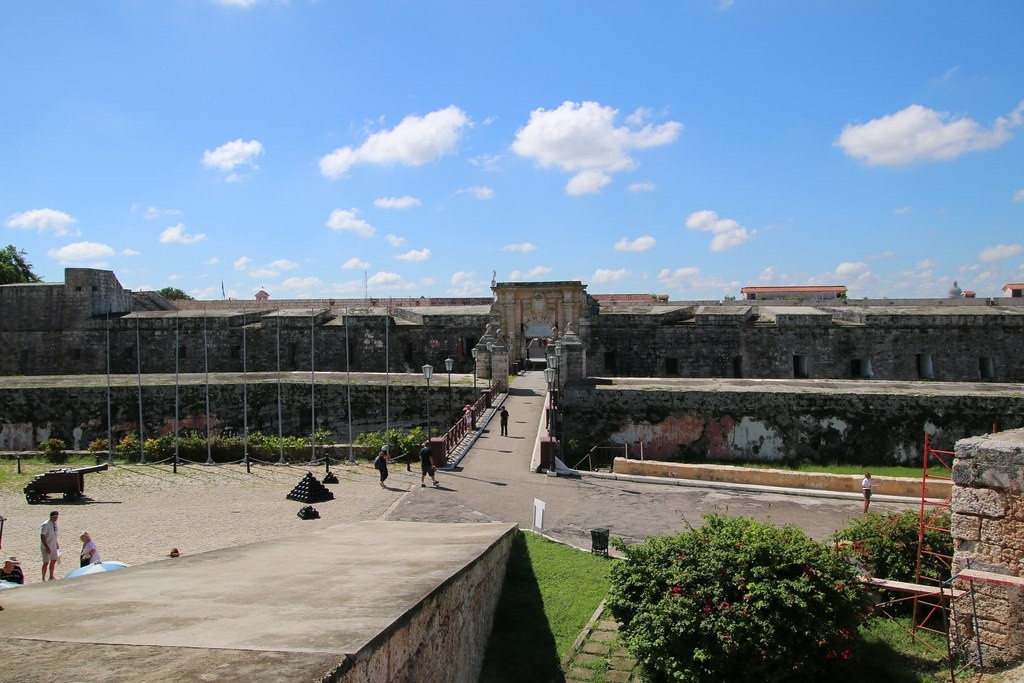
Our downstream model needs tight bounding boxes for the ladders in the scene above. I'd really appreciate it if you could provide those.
[938,558,984,683]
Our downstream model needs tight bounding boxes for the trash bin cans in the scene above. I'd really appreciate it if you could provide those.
[590,528,610,550]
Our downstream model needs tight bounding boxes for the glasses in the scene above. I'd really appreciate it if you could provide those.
[53,526,56,532]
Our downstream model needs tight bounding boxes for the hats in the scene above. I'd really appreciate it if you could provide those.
[466,405,470,408]
[381,446,387,450]
[5,556,21,565]
[167,548,183,557]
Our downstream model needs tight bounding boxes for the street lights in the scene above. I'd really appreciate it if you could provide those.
[554,340,561,398]
[547,355,558,436]
[422,362,434,442]
[486,341,492,389]
[445,355,453,430]
[471,347,478,404]
[544,367,557,476]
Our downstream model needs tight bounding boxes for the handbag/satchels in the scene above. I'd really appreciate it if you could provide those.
[80,555,91,568]
[375,456,382,469]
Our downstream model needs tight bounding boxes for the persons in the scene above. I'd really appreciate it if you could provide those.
[167,548,182,558]
[862,473,876,514]
[465,405,473,434]
[419,442,440,487]
[0,556,24,584]
[40,511,60,582]
[500,406,509,437]
[80,531,102,565]
[379,445,388,487]
[544,350,547,360]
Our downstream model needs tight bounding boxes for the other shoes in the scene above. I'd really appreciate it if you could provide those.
[500,435,503,436]
[421,484,426,487]
[433,480,439,485]
[506,434,507,437]
[467,432,472,434]
[380,482,385,488]
